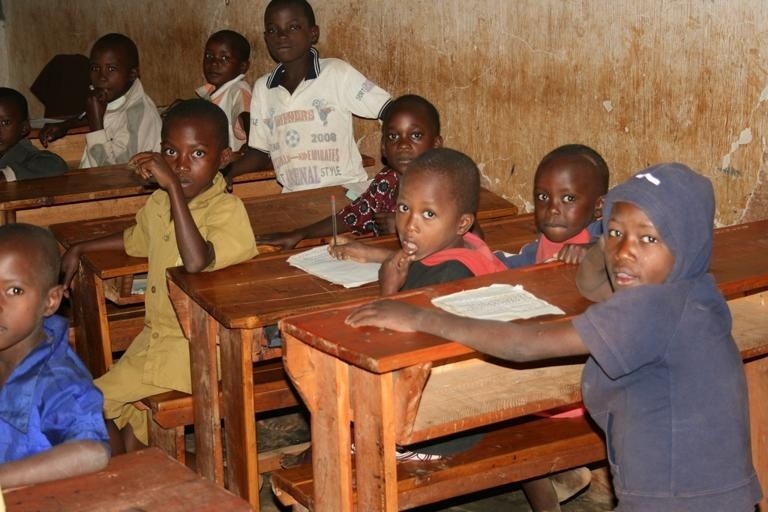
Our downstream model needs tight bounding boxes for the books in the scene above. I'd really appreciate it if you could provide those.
[286,242,386,290]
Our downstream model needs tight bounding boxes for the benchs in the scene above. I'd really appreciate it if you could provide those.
[265,410,606,512]
[65,305,144,364]
[130,361,303,464]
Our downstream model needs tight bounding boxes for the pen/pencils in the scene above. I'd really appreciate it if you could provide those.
[331,193,340,262]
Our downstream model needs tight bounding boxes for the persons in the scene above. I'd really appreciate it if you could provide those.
[281,147,509,512]
[62,99,259,455]
[344,164,763,512]
[220,0,393,193]
[0,87,69,184]
[255,94,486,251]
[0,222,112,491]
[160,30,252,156]
[493,144,608,512]
[38,33,163,169]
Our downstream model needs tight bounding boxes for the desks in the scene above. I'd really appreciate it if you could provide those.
[171,215,550,512]
[273,222,768,512]
[30,111,92,171]
[1,448,261,512]
[1,153,376,226]
[53,189,516,379]
